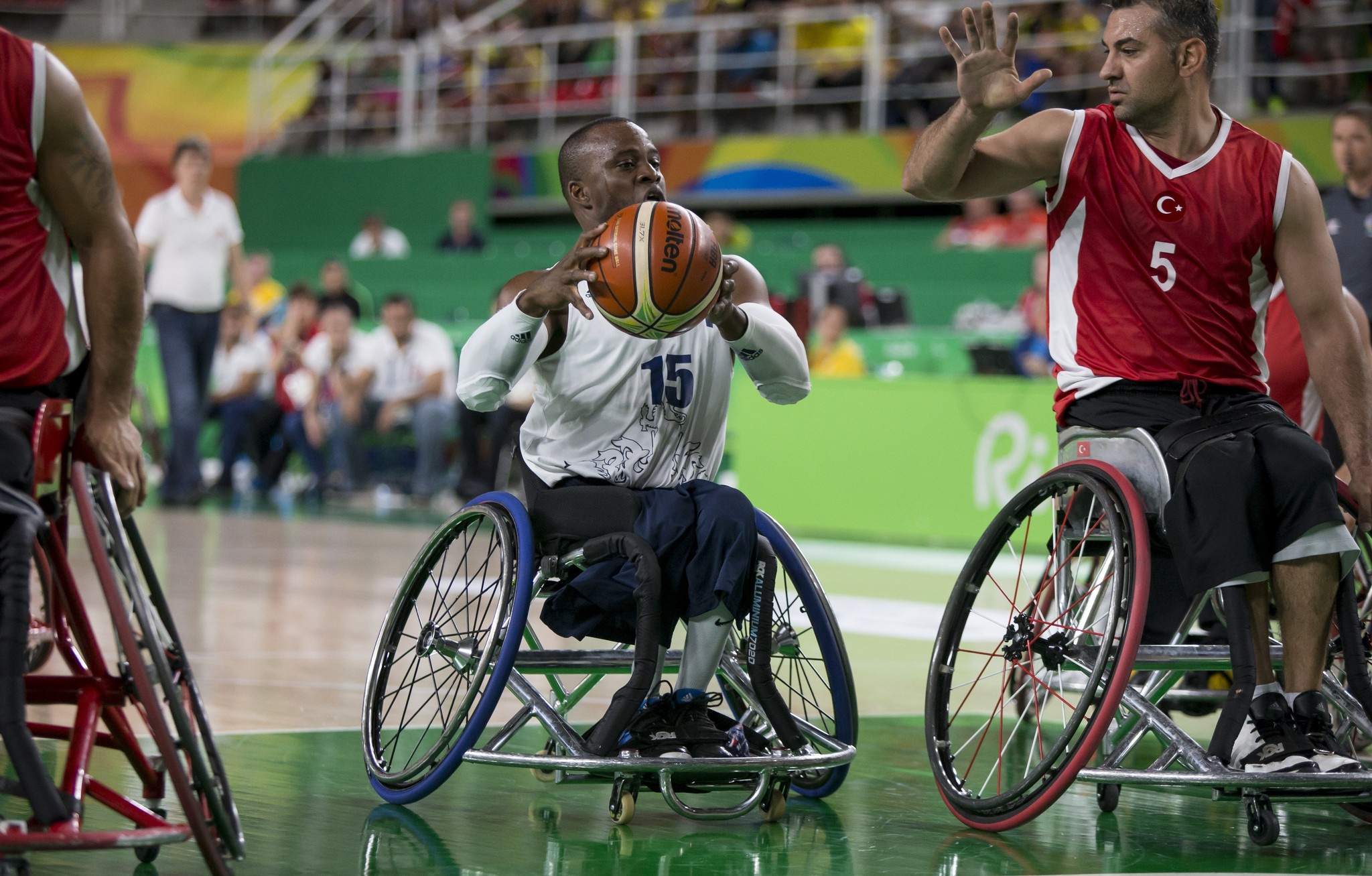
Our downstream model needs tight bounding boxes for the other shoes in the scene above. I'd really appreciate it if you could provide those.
[156,454,468,519]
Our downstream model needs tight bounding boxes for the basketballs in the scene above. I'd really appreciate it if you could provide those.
[585,200,724,341]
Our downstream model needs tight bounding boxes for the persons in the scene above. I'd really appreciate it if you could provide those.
[204,0,1372,647]
[901,0,1371,778]
[456,117,811,759]
[133,137,251,509]
[0,27,151,518]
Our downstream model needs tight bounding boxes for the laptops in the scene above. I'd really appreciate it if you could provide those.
[969,346,1026,375]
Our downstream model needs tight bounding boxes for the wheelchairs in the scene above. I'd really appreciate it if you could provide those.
[1,350,249,876]
[920,426,1372,849]
[360,490,859,826]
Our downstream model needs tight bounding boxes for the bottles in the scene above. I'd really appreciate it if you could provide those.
[234,456,259,518]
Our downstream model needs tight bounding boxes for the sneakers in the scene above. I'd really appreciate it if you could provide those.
[1228,691,1322,775]
[1290,689,1364,772]
[616,679,735,759]
